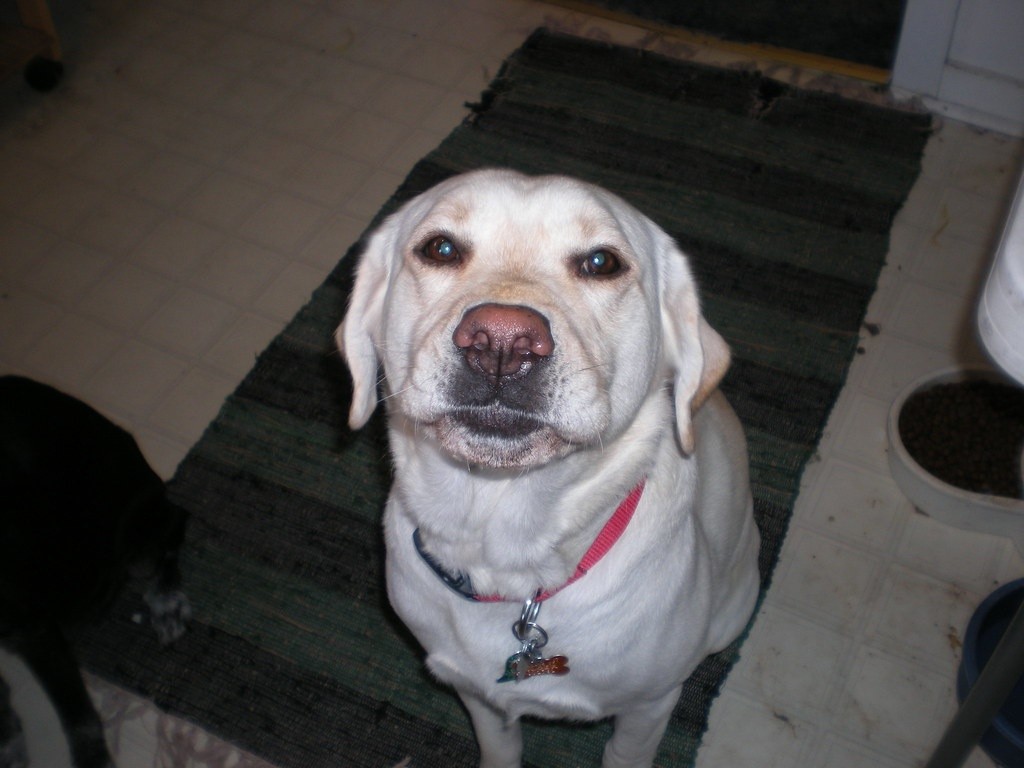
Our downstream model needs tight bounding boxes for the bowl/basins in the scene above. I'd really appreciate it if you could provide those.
[887,364,1023,541]
[957,576,1024,768]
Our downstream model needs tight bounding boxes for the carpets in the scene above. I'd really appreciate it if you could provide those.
[88,22,934,767]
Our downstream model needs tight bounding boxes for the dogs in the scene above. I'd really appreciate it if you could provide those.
[328,163,764,768]
